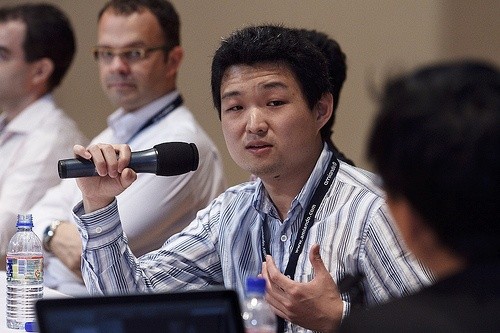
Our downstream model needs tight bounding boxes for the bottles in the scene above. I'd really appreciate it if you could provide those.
[6,214,45,330]
[242,276,277,333]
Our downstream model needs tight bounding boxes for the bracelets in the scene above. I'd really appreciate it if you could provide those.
[339,292,353,323]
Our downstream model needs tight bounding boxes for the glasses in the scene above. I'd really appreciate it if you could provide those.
[91,46,164,61]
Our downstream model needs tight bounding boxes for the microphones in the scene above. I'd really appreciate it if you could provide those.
[58,142,199,179]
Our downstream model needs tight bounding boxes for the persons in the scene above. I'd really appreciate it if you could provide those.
[332,55,500,332]
[21,0,227,288]
[0,1,91,272]
[58,22,439,333]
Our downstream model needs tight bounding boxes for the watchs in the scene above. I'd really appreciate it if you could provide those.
[41,220,65,254]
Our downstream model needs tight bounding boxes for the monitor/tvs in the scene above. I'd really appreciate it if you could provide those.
[34,289,246,333]
